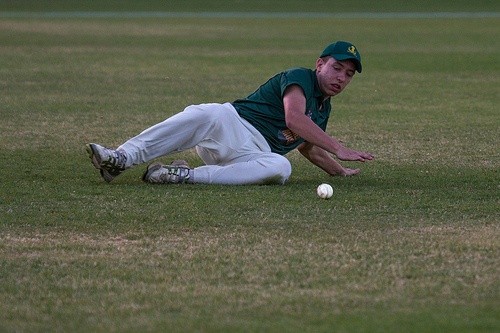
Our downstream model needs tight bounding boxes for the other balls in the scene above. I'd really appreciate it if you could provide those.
[316,183,333,199]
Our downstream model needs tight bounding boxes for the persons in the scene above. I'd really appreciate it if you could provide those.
[86,40,374,186]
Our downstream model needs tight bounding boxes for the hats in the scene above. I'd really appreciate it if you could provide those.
[320,40,362,73]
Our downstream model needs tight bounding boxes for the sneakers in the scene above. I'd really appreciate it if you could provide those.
[85,141,126,182]
[141,159,191,183]
